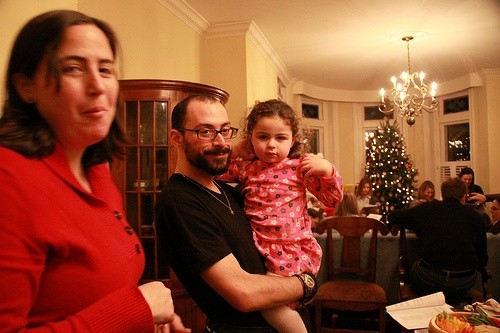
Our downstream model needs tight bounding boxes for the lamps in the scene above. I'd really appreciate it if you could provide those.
[378,36,439,126]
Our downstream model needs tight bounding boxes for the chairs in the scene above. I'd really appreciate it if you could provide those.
[314,218,388,333]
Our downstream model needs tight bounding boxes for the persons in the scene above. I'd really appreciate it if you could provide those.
[305,152,336,231]
[0,8,191,333]
[490,195,500,236]
[335,192,361,217]
[457,167,487,212]
[354,177,380,218]
[214,98,344,333]
[388,177,493,303]
[155,92,321,333]
[413,180,440,207]
[466,192,500,206]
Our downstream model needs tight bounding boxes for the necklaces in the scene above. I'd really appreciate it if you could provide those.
[174,170,235,216]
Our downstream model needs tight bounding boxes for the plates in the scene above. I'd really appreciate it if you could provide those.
[429,312,480,333]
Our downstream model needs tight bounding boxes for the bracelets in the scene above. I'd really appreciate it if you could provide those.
[292,270,319,303]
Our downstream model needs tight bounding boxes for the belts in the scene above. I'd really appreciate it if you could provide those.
[419,260,476,279]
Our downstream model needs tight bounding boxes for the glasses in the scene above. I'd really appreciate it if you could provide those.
[177,126,239,139]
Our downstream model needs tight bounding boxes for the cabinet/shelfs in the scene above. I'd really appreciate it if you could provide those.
[111,79,230,333]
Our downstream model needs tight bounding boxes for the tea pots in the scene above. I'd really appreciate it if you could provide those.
[128,179,149,191]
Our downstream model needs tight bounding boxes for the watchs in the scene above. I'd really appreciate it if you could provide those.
[299,273,316,301]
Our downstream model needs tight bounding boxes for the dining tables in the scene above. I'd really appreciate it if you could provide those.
[411,308,500,333]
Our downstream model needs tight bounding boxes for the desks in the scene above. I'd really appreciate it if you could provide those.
[312,228,500,304]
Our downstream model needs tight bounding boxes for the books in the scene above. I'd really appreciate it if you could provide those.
[384,291,456,331]
[361,202,381,213]
